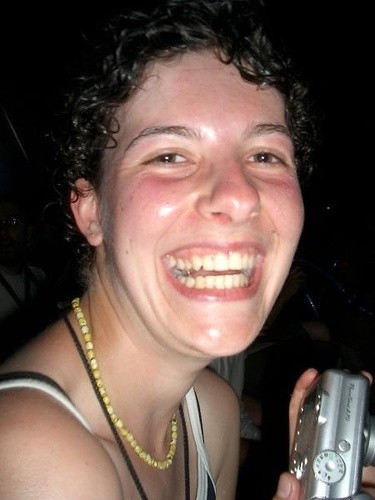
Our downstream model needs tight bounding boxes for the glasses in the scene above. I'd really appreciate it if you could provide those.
[0,216,22,229]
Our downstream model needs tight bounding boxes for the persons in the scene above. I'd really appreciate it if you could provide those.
[1,194,47,317]
[238,264,307,500]
[0,0,375,500]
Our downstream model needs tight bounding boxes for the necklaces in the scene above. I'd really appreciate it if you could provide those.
[62,297,191,500]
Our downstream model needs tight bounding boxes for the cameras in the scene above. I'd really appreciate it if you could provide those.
[288,369,375,500]
